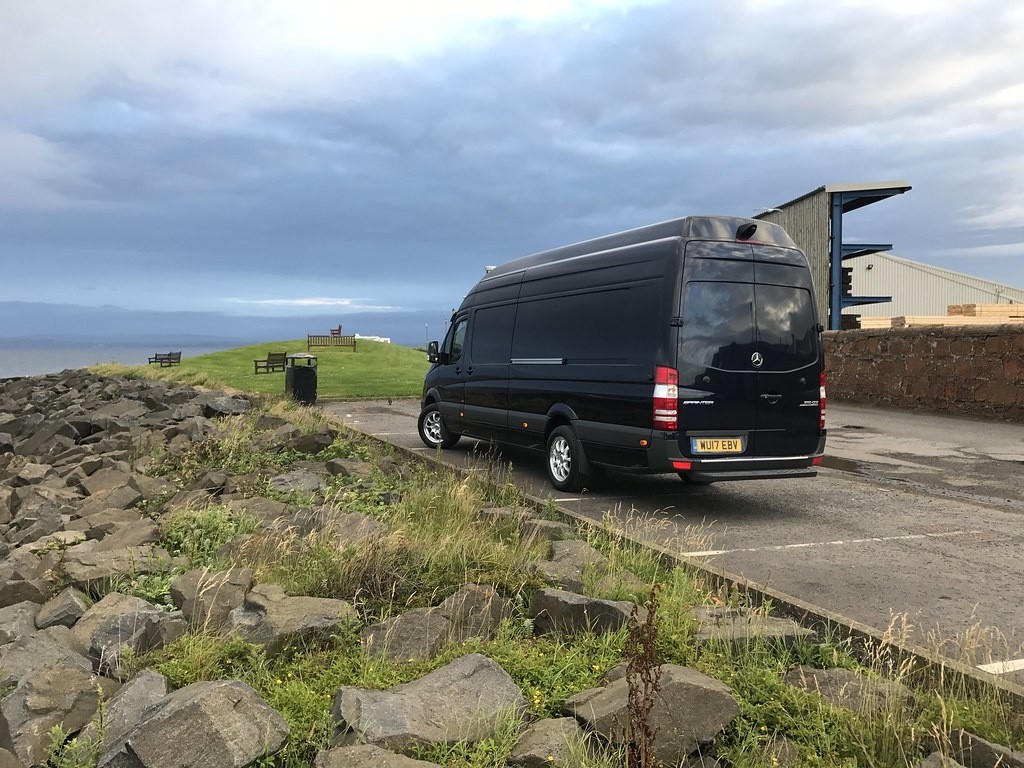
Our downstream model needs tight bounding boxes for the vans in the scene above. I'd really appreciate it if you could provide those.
[416,216,830,492]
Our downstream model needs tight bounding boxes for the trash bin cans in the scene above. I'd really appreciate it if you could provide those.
[285,353,318,407]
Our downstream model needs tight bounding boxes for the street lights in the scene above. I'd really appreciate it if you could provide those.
[445,318,448,336]
[767,208,788,231]
[425,322,429,353]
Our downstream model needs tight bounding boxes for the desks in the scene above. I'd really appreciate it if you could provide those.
[286,354,317,366]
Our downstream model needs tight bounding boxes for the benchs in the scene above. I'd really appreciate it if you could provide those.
[308,334,356,353]
[254,351,286,374]
[148,351,181,367]
